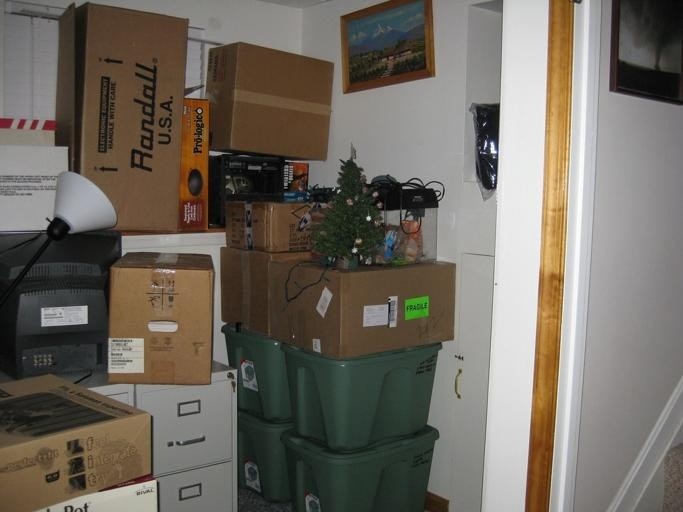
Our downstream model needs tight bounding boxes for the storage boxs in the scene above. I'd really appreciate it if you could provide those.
[55,2,456,512]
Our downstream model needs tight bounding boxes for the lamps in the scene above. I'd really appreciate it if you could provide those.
[0,172,118,326]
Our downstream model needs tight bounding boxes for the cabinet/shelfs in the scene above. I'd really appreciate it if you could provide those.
[427,254,496,512]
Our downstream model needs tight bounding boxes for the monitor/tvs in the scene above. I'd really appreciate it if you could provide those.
[1,231,127,386]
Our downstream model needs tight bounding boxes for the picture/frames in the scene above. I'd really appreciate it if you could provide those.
[610,0,683,105]
